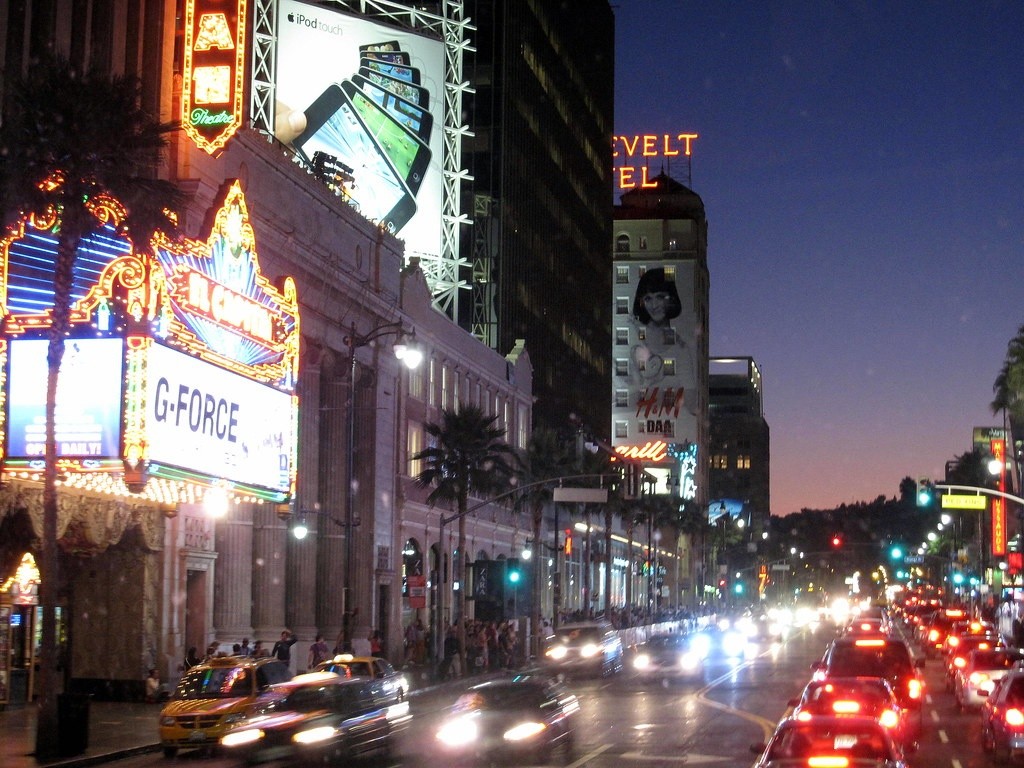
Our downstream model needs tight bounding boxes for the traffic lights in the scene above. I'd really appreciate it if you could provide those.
[916,476,932,506]
[735,584,743,593]
[833,538,840,545]
[892,549,902,558]
[509,572,520,583]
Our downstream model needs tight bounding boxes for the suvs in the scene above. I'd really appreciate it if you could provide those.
[979,659,1024,768]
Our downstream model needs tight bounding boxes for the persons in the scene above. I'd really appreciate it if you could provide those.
[630,267,697,412]
[271,630,298,668]
[273,101,306,161]
[533,603,722,639]
[307,634,333,670]
[333,629,382,657]
[144,670,170,709]
[401,617,518,680]
[176,638,269,680]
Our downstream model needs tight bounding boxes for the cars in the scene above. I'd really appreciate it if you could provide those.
[309,654,409,704]
[543,620,623,678]
[435,676,571,768]
[158,658,292,763]
[635,632,704,683]
[943,632,1009,693]
[810,637,925,731]
[835,591,995,665]
[789,678,910,752]
[216,670,415,768]
[749,715,908,768]
[952,647,1013,714]
